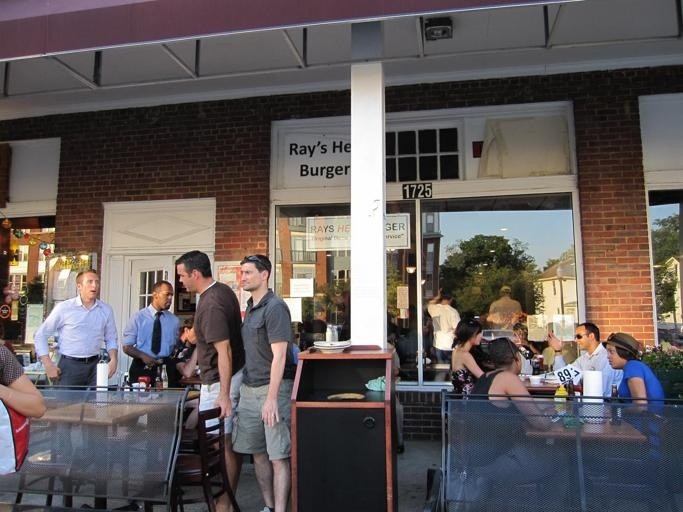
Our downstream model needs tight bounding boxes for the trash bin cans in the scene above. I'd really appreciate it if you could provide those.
[289,354,395,512]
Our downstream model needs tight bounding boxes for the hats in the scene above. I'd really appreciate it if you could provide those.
[602,332,641,361]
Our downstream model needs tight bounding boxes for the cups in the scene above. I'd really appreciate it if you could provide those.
[529,375,541,388]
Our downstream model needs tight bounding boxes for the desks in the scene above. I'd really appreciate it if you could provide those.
[520,414,648,509]
[526,379,582,394]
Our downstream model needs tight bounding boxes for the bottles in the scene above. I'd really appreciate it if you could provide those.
[120,363,169,402]
[609,382,621,426]
[551,379,578,423]
[325,323,338,342]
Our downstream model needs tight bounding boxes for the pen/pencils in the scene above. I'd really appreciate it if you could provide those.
[544,370,547,379]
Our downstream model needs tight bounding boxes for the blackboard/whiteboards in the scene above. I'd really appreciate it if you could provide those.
[24,303,44,342]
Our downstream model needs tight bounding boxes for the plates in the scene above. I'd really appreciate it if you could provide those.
[313,340,351,355]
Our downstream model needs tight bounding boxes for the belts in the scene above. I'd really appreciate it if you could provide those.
[61,354,99,365]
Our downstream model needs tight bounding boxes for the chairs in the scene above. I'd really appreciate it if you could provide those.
[12,377,240,512]
[449,411,545,507]
[587,410,670,508]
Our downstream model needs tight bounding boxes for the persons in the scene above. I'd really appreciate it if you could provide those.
[0,344,47,419]
[230,254,296,512]
[169,319,199,429]
[122,281,181,391]
[33,269,118,453]
[175,250,245,512]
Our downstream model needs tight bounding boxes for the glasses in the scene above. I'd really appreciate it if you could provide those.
[575,333,589,339]
[247,256,269,270]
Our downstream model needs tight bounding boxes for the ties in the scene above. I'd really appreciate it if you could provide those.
[151,312,163,356]
